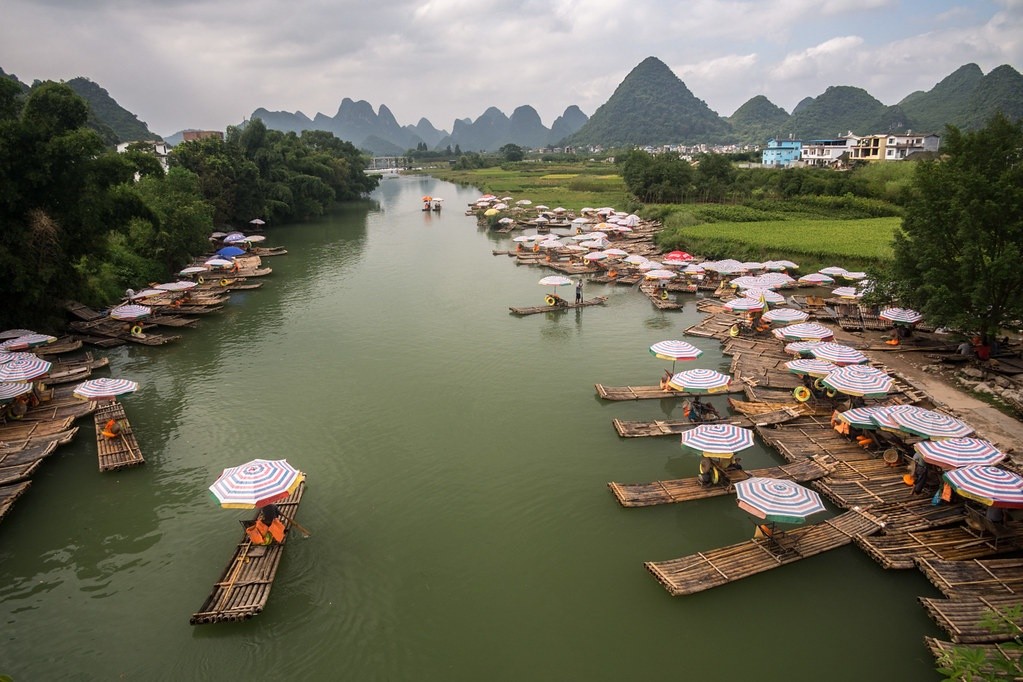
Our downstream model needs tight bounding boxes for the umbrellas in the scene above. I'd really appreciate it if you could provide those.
[422,196,444,202]
[208,458,304,509]
[111,304,151,325]
[73,377,138,416]
[0,329,58,399]
[181,219,266,275]
[840,405,1023,508]
[539,276,574,295]
[478,195,672,279]
[130,281,198,300]
[663,250,926,398]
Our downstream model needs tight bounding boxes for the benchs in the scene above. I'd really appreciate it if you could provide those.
[546,293,568,308]
[239,519,273,538]
[666,295,1019,568]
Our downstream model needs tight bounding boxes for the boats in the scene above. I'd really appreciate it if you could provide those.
[185,472,307,629]
[419,192,1022,682]
[0,220,287,523]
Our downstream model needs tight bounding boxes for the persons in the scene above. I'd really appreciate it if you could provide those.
[97,417,119,437]
[682,425,754,463]
[957,340,969,354]
[734,477,828,537]
[987,508,1002,522]
[693,396,718,415]
[576,279,583,304]
[263,503,280,526]
[659,284,667,292]
[425,203,437,209]
[973,342,992,363]
[991,336,1001,354]
[125,287,137,304]
